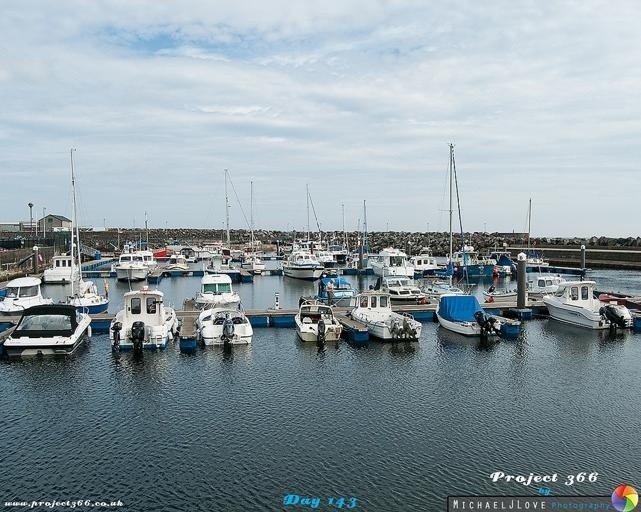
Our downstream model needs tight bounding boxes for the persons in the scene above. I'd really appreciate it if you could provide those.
[327,278,336,306]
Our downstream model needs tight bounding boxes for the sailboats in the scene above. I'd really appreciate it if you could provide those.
[525,198,550,267]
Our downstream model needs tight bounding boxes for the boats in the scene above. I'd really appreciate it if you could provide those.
[4,304,94,356]
[116,254,149,282]
[544,280,634,330]
[242,180,266,270]
[165,254,190,278]
[1,277,54,315]
[57,146,110,314]
[195,273,240,309]
[435,294,501,336]
[351,290,422,341]
[371,247,414,278]
[315,276,355,307]
[198,308,253,346]
[484,285,529,302]
[295,304,344,341]
[135,251,159,269]
[409,253,446,280]
[109,287,178,352]
[180,248,196,262]
[527,273,567,300]
[284,253,324,280]
[426,143,466,301]
[318,200,370,266]
[368,267,425,306]
[456,250,515,268]
[206,255,240,283]
[43,253,80,283]
